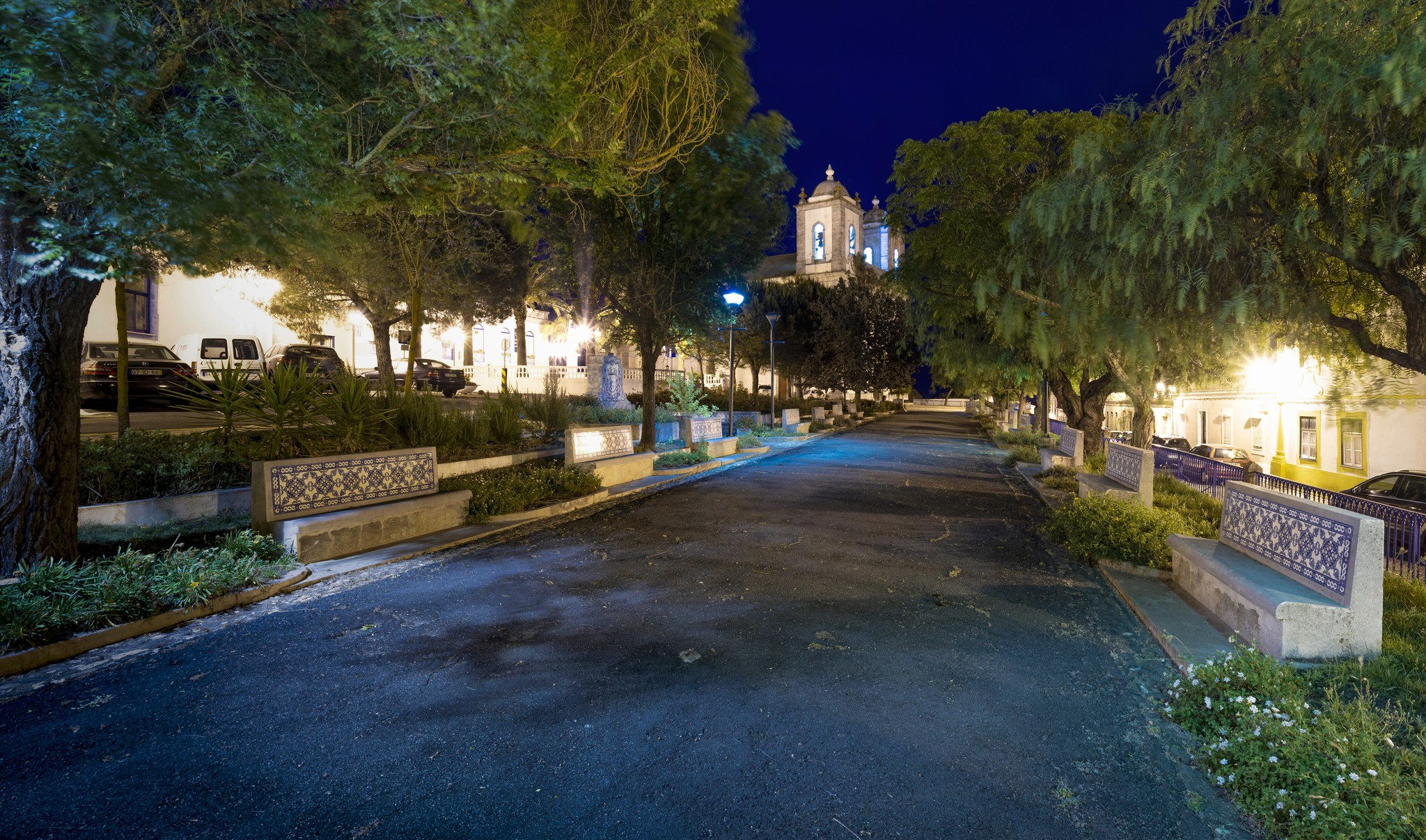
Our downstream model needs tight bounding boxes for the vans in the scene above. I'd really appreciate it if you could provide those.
[171,331,265,395]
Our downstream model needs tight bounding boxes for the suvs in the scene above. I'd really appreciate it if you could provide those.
[1151,433,1190,465]
[1329,470,1426,566]
[264,343,346,395]
[758,384,771,396]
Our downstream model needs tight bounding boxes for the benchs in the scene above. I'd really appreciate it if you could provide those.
[565,425,654,486]
[251,447,473,564]
[782,403,864,434]
[685,416,739,458]
[980,405,1154,512]
[1166,480,1384,670]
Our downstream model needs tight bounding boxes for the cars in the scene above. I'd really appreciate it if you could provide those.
[803,390,825,400]
[1102,431,1132,444]
[347,358,465,397]
[79,340,193,410]
[1180,444,1251,484]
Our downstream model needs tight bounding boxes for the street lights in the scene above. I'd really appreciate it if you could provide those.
[760,313,784,431]
[717,290,748,437]
